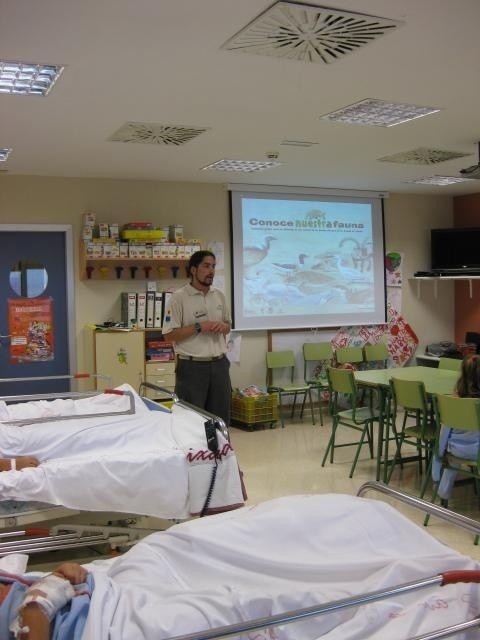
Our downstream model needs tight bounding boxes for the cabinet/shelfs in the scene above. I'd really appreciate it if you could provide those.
[93,327,177,400]
[411,276,480,300]
[78,236,203,279]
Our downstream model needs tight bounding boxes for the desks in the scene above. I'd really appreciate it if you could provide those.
[416,353,440,366]
[353,365,480,488]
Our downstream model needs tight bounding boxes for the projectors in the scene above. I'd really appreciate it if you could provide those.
[459,163,480,180]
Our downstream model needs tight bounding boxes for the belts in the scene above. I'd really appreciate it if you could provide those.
[177,354,225,362]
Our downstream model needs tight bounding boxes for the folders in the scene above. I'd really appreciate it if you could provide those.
[136,292,146,329]
[164,291,173,315]
[153,292,162,328]
[146,291,155,328]
[127,291,137,329]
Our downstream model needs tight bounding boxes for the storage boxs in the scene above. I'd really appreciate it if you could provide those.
[231,390,278,432]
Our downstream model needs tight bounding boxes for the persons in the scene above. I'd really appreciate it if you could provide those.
[0,455,40,472]
[161,250,232,431]
[0,562,95,640]
[226,340,234,362]
[432,353,480,499]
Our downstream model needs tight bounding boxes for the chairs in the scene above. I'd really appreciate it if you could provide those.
[423,390,480,545]
[266,350,316,428]
[336,346,368,413]
[303,342,344,426]
[438,358,464,371]
[385,376,438,488]
[364,343,388,407]
[321,365,403,478]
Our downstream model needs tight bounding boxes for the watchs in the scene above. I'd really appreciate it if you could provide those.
[194,322,201,333]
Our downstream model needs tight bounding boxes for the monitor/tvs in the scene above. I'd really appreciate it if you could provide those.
[429,225,480,276]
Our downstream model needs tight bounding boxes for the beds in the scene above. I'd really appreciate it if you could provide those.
[0,373,248,559]
[1,481,480,639]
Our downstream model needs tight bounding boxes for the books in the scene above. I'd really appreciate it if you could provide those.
[121,290,173,329]
[146,340,175,363]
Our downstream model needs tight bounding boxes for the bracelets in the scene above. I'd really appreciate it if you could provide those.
[11,458,16,470]
